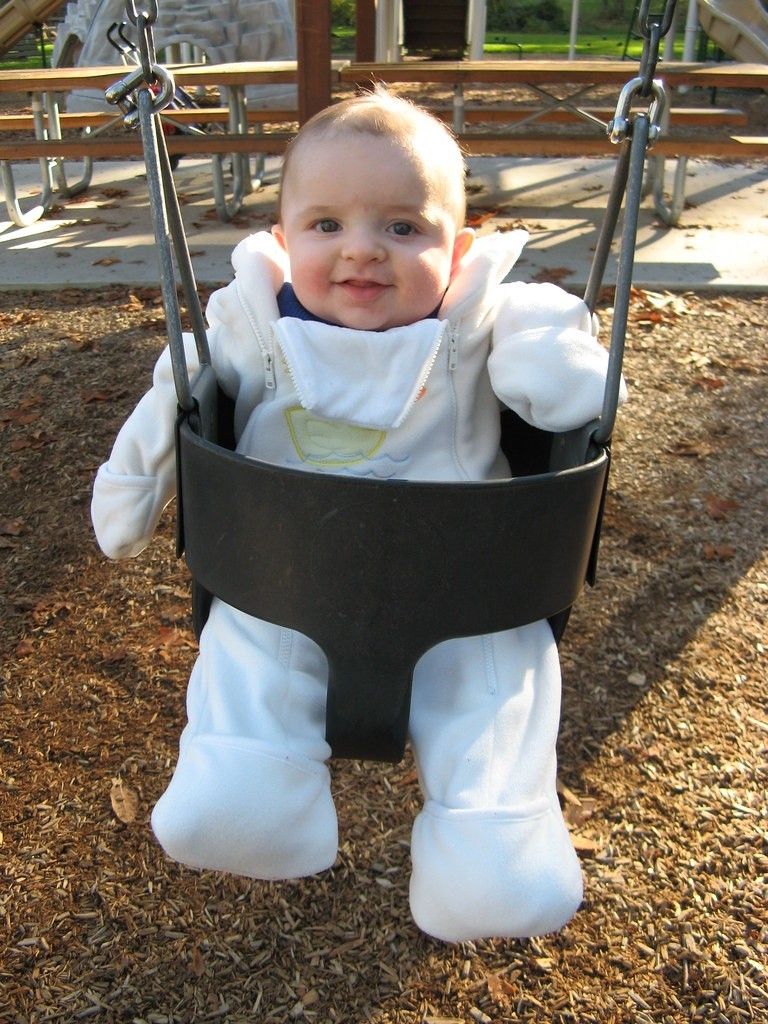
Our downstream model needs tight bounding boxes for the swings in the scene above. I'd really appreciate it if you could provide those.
[108,0,679,769]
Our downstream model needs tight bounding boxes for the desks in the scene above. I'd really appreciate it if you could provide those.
[0,58,768,228]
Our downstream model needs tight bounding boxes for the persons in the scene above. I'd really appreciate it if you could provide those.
[90,93,626,941]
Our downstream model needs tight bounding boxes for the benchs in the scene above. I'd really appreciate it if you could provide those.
[0,108,768,228]
